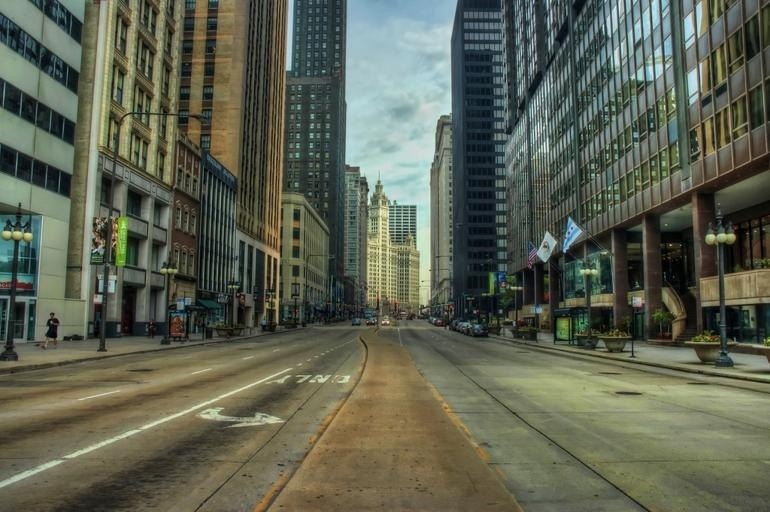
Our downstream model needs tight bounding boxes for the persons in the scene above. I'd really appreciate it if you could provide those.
[147,320,156,338]
[40,311,60,350]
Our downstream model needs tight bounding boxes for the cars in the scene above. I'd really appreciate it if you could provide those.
[449,320,489,336]
[351,317,361,326]
[381,318,390,326]
[365,317,375,326]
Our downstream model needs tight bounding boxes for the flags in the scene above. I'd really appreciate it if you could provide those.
[481,270,507,297]
[563,217,584,254]
[536,231,558,263]
[115,216,129,267]
[527,242,538,270]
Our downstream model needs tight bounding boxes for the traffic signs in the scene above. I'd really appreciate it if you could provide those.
[501,274,506,337]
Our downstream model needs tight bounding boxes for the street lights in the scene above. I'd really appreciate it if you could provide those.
[510,276,523,325]
[227,277,240,328]
[579,256,600,350]
[301,254,336,328]
[704,200,737,366]
[96,112,210,352]
[0,202,33,361]
[160,251,179,346]
